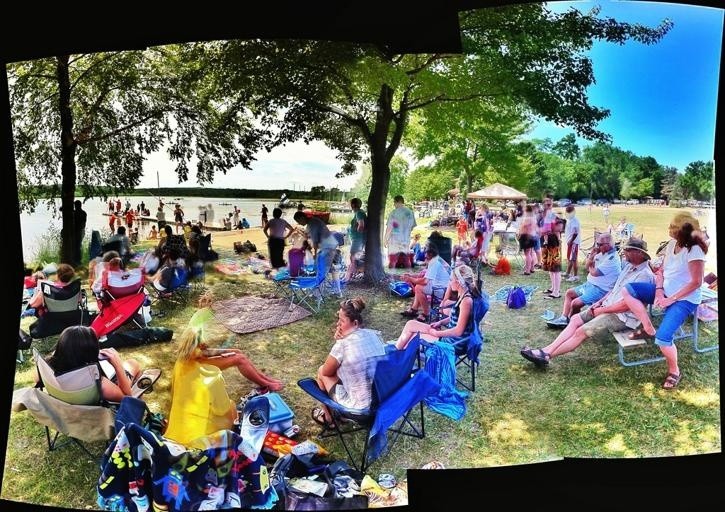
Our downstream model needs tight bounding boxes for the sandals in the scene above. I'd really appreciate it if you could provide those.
[628,328,656,338]
[417,313,430,321]
[520,349,551,364]
[401,308,418,316]
[544,272,580,299]
[312,407,335,431]
[662,373,682,390]
[521,264,541,275]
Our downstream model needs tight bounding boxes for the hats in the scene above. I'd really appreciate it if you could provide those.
[618,237,651,260]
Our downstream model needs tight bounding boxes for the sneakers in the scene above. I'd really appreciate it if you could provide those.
[546,314,568,328]
[333,277,346,299]
[236,387,269,410]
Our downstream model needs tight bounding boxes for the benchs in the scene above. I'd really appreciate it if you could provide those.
[690,285,719,353]
[612,328,668,367]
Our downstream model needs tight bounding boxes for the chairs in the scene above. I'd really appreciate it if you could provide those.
[502,237,526,269]
[581,229,611,257]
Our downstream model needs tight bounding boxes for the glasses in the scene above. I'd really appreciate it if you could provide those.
[623,248,639,251]
[597,242,609,246]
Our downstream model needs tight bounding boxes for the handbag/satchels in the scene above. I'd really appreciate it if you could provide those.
[506,287,525,308]
[288,248,305,276]
[269,453,368,511]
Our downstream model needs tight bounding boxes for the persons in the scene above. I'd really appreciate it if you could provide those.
[621,211,708,390]
[395,263,476,355]
[546,233,622,330]
[35,325,142,402]
[174,327,285,391]
[311,298,387,431]
[520,237,656,365]
[30,196,627,323]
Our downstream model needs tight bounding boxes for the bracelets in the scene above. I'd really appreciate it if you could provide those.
[671,294,677,300]
[655,287,664,290]
